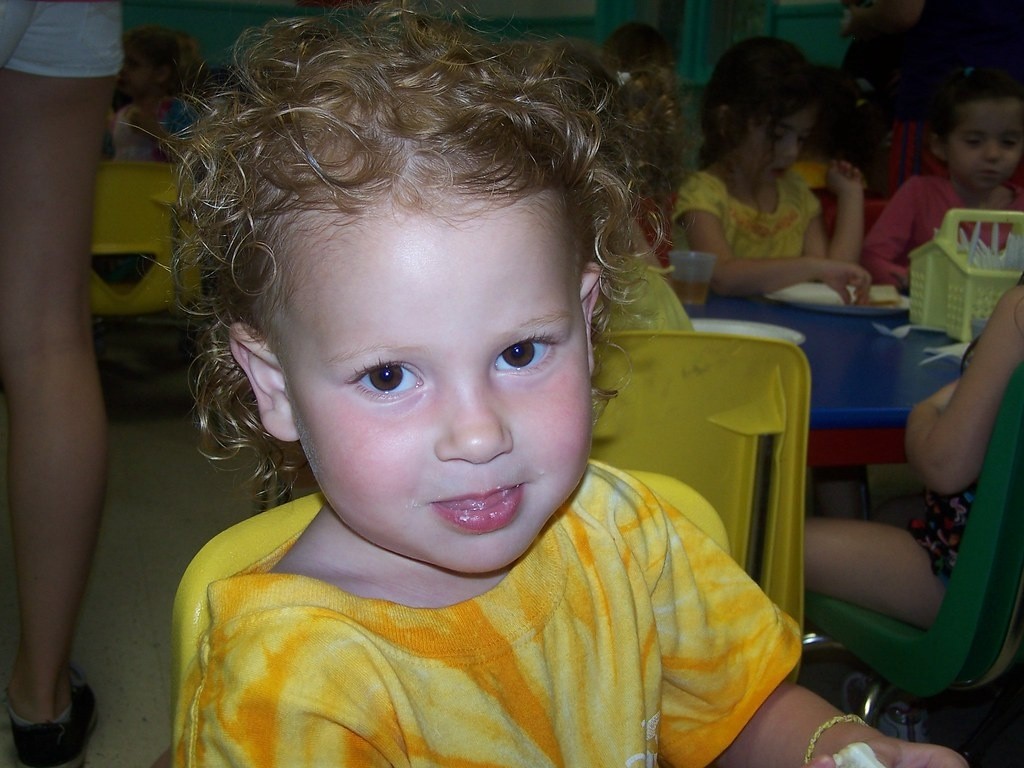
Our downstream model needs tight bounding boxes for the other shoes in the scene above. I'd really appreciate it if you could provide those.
[9,670,97,768]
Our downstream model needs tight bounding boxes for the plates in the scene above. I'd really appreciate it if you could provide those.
[689,319,807,347]
[763,282,910,316]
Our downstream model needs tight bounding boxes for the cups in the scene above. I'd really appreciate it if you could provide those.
[667,250,717,308]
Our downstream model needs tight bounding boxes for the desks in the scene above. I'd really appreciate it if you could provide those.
[674,288,993,472]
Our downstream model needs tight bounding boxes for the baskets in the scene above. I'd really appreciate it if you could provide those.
[910,209,1024,343]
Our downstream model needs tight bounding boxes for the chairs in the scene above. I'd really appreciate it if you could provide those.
[586,328,811,685]
[86,157,209,342]
[796,352,1024,739]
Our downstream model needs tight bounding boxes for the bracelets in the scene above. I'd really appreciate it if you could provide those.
[804,715,869,764]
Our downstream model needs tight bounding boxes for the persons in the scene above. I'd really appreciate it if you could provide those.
[803,271,1024,768]
[117,0,1024,520]
[176,0,969,768]
[0,0,124,768]
